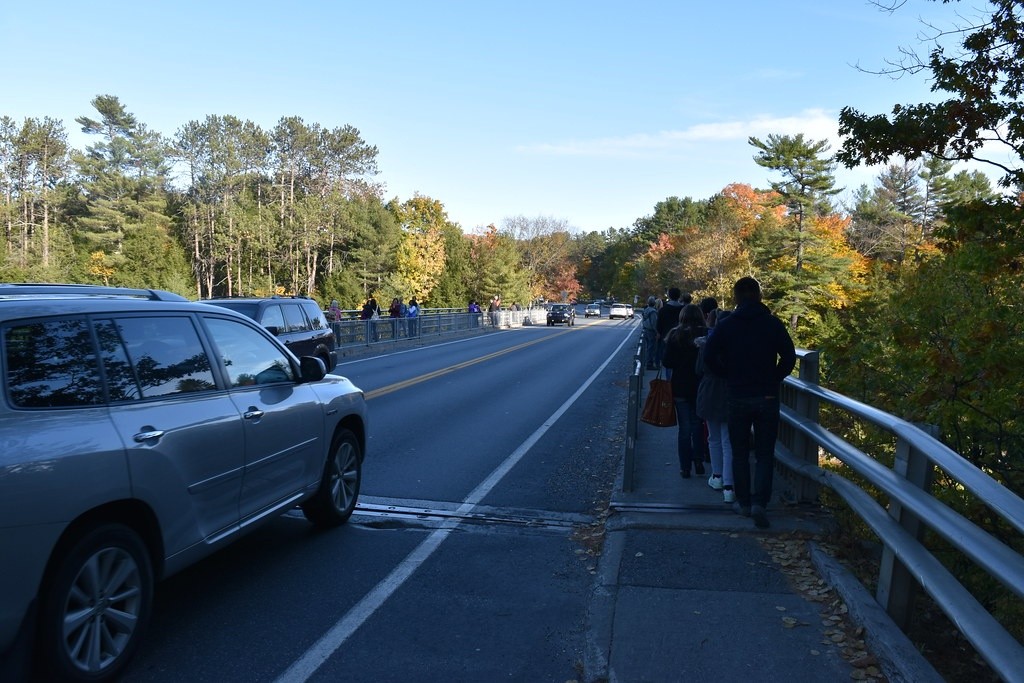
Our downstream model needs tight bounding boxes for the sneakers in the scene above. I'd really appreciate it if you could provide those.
[707,474,723,491]
[722,488,736,504]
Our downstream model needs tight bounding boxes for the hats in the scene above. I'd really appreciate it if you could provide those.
[647,295,656,304]
[331,299,338,308]
[700,297,718,313]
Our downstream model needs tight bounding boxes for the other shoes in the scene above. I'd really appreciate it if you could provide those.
[695,462,705,475]
[750,504,769,529]
[731,500,749,516]
[679,469,690,479]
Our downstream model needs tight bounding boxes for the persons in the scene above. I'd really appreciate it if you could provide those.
[703,277,795,532]
[388,295,420,337]
[363,298,381,342]
[468,293,522,328]
[642,288,737,504]
[327,299,342,347]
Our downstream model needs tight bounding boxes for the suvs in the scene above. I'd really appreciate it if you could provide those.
[191,294,340,375]
[0,282,369,683]
[546,304,575,327]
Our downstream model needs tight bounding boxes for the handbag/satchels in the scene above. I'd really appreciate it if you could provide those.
[639,360,678,427]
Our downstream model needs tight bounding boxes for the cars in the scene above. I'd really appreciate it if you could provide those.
[593,301,635,320]
[584,304,602,318]
[570,299,578,305]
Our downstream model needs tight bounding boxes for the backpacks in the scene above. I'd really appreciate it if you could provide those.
[370,305,380,323]
